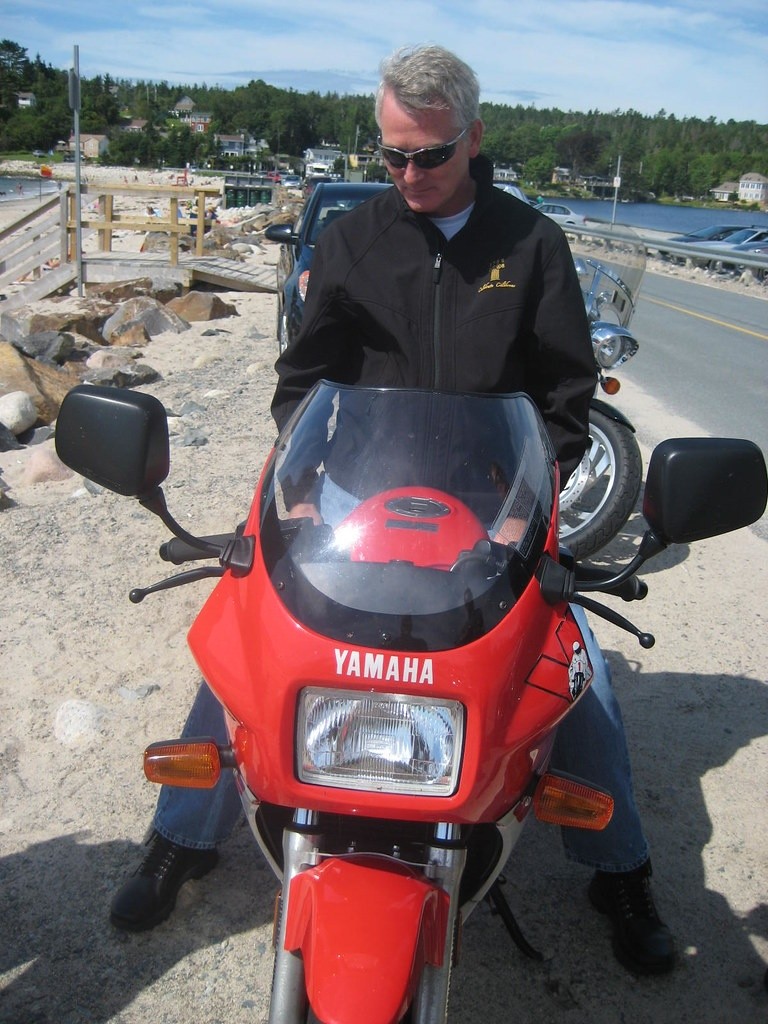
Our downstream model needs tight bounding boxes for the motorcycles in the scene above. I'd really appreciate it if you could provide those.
[52,380,767,1024]
[547,217,650,563]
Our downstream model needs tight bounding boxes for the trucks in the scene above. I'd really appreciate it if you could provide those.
[305,163,330,176]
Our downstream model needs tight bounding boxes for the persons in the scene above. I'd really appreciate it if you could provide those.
[56,180,63,190]
[16,183,25,196]
[108,45,679,978]
[189,199,218,235]
[145,204,168,236]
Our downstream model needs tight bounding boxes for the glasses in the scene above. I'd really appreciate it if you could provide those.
[376,125,468,168]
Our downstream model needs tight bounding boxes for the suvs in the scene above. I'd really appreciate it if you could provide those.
[300,172,333,203]
[62,149,89,163]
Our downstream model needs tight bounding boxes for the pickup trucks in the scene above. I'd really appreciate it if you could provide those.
[32,149,46,159]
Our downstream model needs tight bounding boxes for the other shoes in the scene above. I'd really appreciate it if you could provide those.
[587,862,676,977]
[110,829,218,931]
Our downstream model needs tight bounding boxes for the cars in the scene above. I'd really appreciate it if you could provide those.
[257,170,301,188]
[490,183,532,206]
[659,223,768,284]
[265,181,395,359]
[534,202,585,227]
